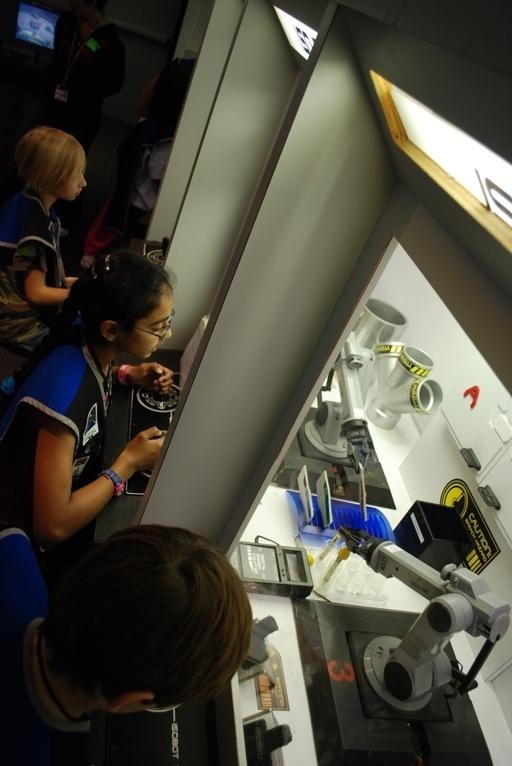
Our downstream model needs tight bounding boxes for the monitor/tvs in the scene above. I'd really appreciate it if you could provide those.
[14,1,60,51]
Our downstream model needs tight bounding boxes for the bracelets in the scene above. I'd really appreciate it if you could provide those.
[116,363,135,387]
[94,468,127,501]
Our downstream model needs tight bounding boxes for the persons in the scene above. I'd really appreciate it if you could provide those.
[0,505,256,765]
[1,248,175,549]
[52,0,127,159]
[83,55,198,254]
[0,122,89,394]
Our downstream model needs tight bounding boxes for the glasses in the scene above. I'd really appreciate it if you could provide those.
[136,308,175,340]
